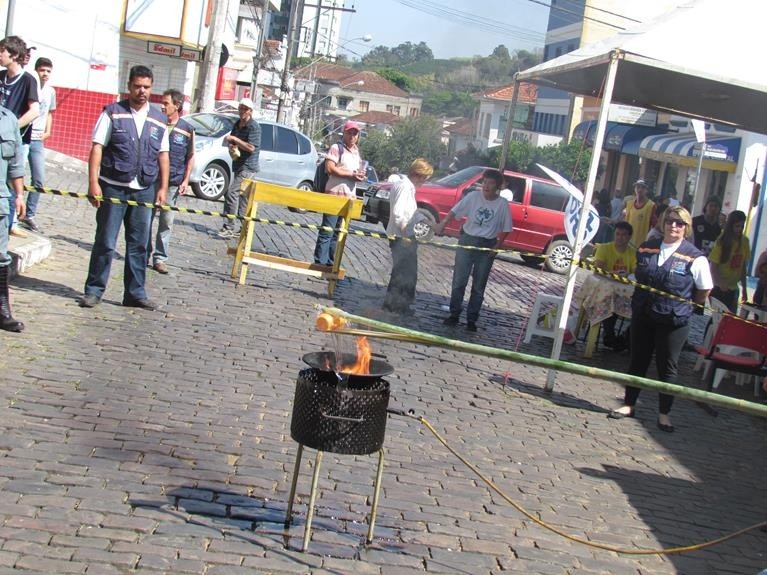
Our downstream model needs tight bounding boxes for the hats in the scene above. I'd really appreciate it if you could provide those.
[594,180,728,219]
[344,121,360,132]
[239,98,253,110]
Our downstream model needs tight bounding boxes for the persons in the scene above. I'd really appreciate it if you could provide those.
[382,157,434,315]
[0,37,41,235]
[0,104,28,331]
[388,166,398,183]
[149,88,196,274]
[313,121,368,268]
[474,178,514,201]
[73,63,172,312]
[19,57,57,231]
[604,204,715,434]
[16,40,43,104]
[214,97,263,236]
[437,167,514,329]
[576,175,767,358]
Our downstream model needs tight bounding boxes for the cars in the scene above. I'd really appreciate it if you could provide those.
[175,113,317,216]
[364,164,588,273]
[352,164,382,217]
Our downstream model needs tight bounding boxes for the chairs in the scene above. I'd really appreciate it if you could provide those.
[693,296,761,396]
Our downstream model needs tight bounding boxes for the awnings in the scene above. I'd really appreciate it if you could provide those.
[573,119,743,174]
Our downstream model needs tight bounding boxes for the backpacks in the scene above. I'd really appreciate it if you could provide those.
[312,161,329,193]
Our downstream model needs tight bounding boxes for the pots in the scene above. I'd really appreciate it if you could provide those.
[301,350,395,388]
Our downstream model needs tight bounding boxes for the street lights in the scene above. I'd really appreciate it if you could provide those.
[277,34,374,146]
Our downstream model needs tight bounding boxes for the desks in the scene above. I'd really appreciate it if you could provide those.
[574,274,637,359]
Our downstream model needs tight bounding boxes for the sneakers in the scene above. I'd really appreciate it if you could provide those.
[657,417,674,432]
[23,216,41,233]
[217,224,232,240]
[609,407,635,419]
[233,232,240,238]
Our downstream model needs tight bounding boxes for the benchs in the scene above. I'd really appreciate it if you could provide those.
[693,312,767,420]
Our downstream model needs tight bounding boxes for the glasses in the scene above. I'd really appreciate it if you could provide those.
[665,218,686,226]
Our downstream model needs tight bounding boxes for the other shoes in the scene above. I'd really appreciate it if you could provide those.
[381,297,636,352]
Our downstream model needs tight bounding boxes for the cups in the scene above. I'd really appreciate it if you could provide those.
[316,306,348,332]
[358,160,368,173]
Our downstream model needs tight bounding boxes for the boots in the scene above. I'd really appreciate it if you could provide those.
[0,254,24,331]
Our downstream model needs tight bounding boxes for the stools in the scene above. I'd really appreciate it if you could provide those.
[522,291,564,345]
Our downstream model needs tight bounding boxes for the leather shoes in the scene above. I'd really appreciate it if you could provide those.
[123,298,160,311]
[153,261,168,274]
[79,294,102,307]
